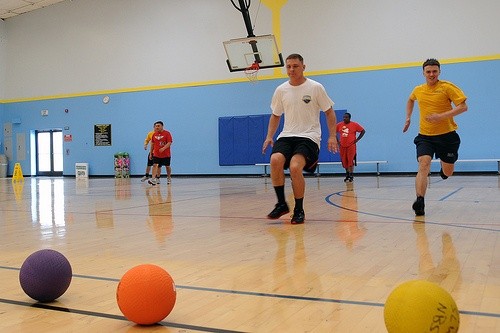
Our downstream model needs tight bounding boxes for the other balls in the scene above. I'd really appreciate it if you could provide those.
[116,264,176,324]
[384,279,460,333]
[20,249,72,302]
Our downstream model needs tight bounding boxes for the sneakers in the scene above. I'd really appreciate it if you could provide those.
[139,175,172,185]
[412,200,426,216]
[268,201,289,219]
[290,207,306,224]
[344,175,354,182]
[440,167,448,178]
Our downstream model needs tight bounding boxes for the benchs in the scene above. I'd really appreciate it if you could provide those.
[255,161,388,177]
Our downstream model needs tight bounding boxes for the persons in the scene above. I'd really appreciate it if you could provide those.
[336,113,366,182]
[141,122,163,184]
[148,121,172,185]
[261,54,339,224]
[403,59,468,216]
